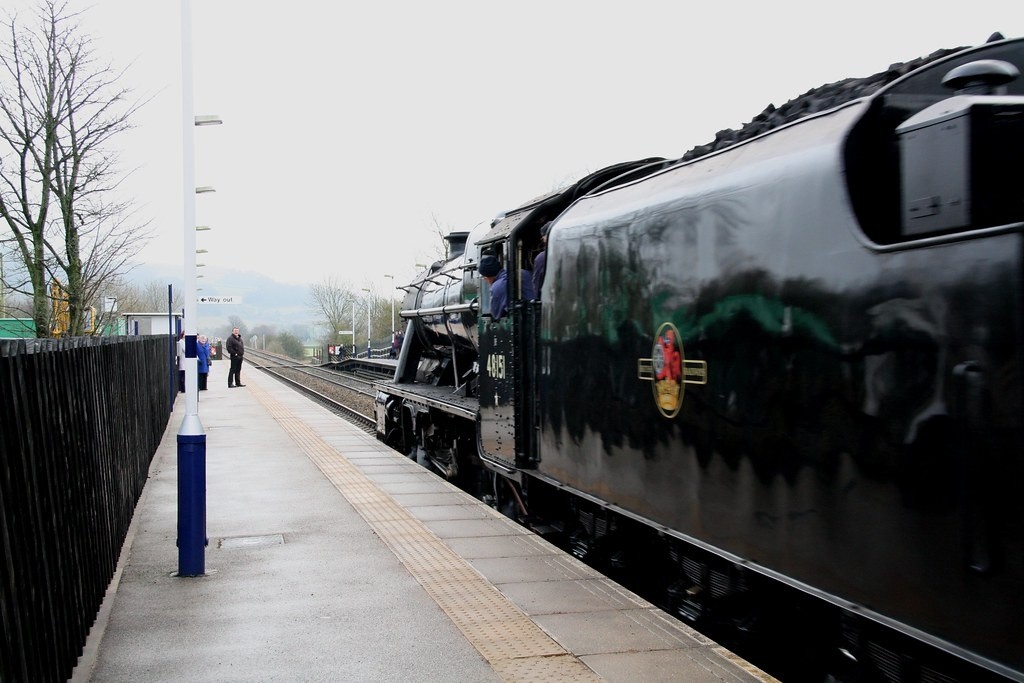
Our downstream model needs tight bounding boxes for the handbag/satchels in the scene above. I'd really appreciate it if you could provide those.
[207,357,212,366]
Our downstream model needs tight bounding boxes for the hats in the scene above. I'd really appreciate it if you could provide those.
[540,221,553,237]
[479,257,502,278]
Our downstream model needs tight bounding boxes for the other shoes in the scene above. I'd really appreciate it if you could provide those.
[228,385,236,388]
[236,383,246,387]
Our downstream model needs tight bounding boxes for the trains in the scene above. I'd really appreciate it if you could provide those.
[371,30,1023,683]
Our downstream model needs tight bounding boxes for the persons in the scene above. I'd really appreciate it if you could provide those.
[225,327,246,388]
[533,219,556,302]
[390,329,404,359]
[196,335,210,391]
[176,331,199,393]
[477,256,533,319]
[337,344,346,361]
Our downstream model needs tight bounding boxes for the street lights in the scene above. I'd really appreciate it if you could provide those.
[384,274,395,356]
[362,288,371,359]
[348,298,356,358]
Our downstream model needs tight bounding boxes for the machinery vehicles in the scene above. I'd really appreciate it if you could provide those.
[46,274,98,336]
